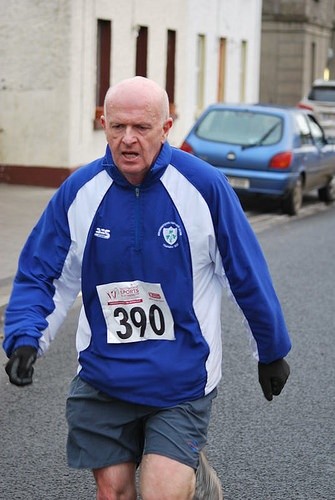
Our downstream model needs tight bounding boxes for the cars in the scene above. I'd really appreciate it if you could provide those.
[177,104,335,218]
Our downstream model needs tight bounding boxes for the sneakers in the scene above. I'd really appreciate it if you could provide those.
[194,450,223,500]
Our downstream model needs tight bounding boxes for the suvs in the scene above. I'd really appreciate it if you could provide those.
[291,80,335,145]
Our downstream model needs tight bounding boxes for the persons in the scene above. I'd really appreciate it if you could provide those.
[3,75,291,500]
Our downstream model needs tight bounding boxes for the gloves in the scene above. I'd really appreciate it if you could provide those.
[5,345,38,387]
[258,357,290,402]
